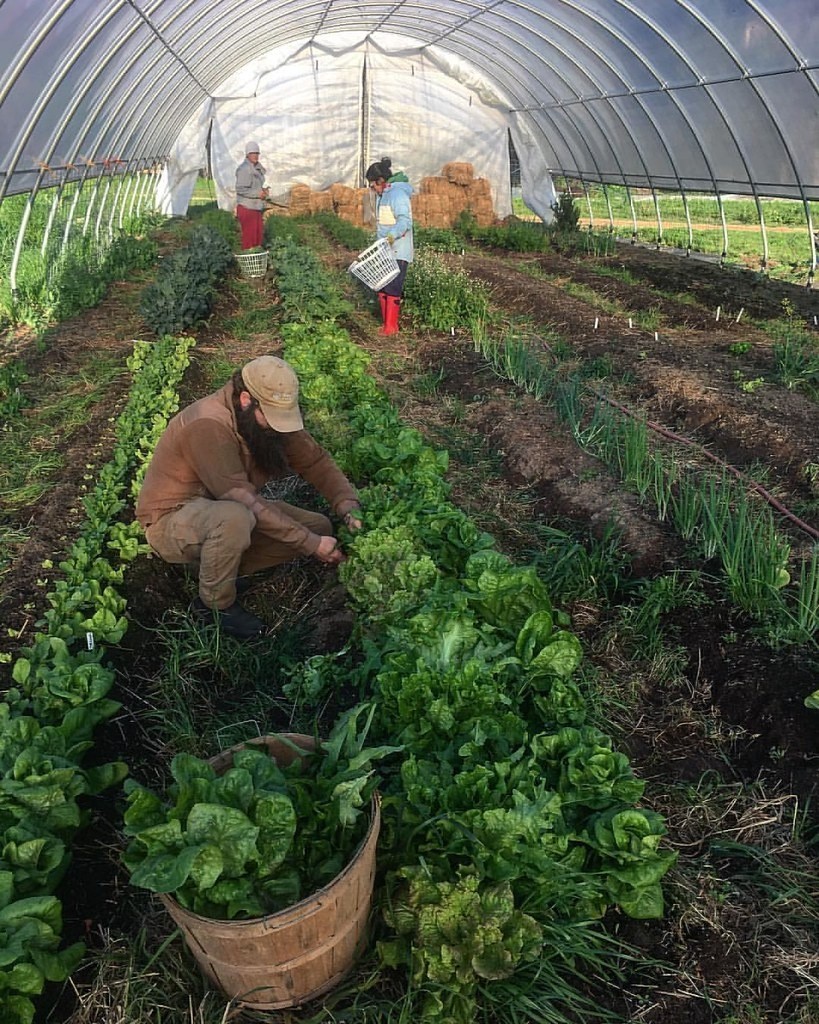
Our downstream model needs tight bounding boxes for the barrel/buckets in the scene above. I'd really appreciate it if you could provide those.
[149,733,383,1011]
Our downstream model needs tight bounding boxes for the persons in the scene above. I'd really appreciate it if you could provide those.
[135,356,363,640]
[235,139,270,252]
[364,157,414,335]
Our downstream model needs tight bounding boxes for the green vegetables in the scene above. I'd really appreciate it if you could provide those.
[120,699,406,920]
[236,246,264,275]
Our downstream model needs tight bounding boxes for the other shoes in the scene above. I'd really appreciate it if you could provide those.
[192,597,267,637]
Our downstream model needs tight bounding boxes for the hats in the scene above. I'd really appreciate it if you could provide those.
[241,355,304,433]
[245,142,260,156]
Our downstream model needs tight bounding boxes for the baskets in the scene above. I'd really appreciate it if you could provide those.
[234,250,268,278]
[349,236,400,292]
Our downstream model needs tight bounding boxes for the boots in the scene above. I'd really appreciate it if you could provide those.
[376,293,401,337]
